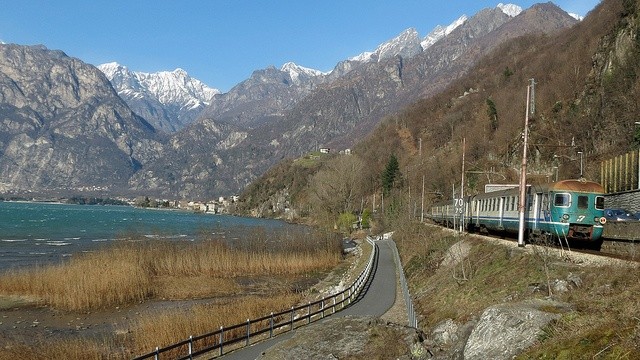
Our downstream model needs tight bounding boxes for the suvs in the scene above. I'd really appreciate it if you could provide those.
[606,209,638,221]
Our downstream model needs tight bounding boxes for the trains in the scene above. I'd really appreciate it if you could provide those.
[431,178,607,240]
